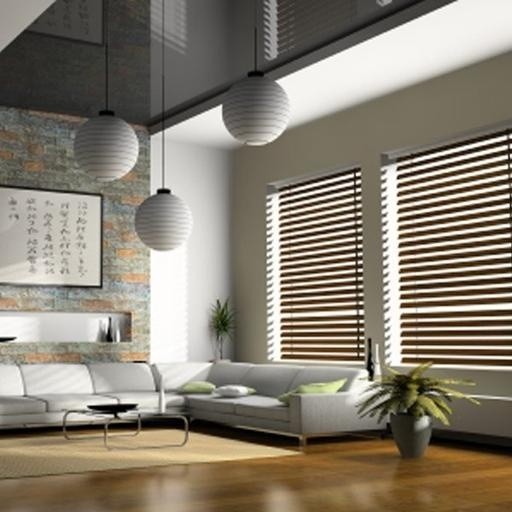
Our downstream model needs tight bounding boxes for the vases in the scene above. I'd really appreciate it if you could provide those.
[373,344,382,375]
[112,320,121,343]
[159,375,166,413]
[107,317,114,342]
[366,338,374,381]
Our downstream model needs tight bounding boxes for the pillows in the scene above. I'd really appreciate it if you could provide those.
[178,381,215,393]
[279,378,348,404]
[212,385,256,397]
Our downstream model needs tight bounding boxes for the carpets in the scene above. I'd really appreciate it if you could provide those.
[0,429,303,478]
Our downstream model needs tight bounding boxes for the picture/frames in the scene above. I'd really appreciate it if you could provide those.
[0,185,103,288]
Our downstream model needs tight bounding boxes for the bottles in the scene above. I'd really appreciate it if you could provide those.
[366,337,382,383]
[105,318,120,343]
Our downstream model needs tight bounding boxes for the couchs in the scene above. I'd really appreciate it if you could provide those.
[0,360,387,446]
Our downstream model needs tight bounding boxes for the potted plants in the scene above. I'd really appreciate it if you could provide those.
[356,362,481,459]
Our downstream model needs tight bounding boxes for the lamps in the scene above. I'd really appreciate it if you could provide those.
[136,2,191,249]
[222,4,290,147]
[77,0,142,181]
